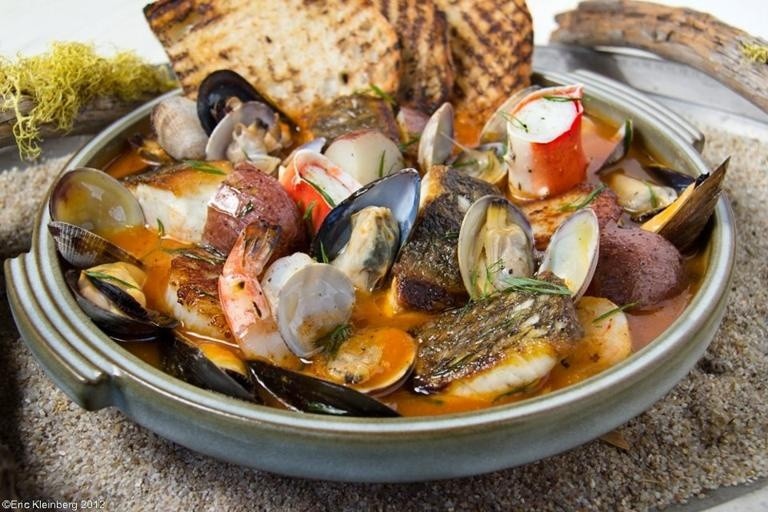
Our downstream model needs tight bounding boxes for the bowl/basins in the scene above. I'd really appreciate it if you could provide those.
[2,63,739,488]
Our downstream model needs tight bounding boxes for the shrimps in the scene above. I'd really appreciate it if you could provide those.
[216,221,305,377]
